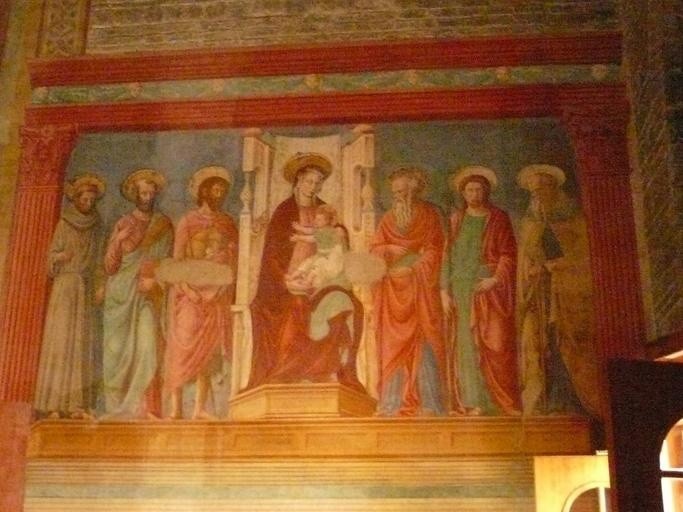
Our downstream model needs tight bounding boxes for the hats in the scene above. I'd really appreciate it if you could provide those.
[448,164,498,193]
[514,164,566,190]
[64,173,107,202]
[188,166,234,199]
[119,168,167,203]
[280,151,333,182]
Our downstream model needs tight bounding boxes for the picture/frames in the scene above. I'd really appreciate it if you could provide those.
[23,0,622,90]
[0,83,645,458]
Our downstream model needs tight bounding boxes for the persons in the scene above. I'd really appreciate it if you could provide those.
[31,176,108,422]
[282,203,348,289]
[366,166,446,417]
[233,152,366,397]
[514,163,606,422]
[436,164,523,418]
[163,166,239,419]
[101,167,172,419]
